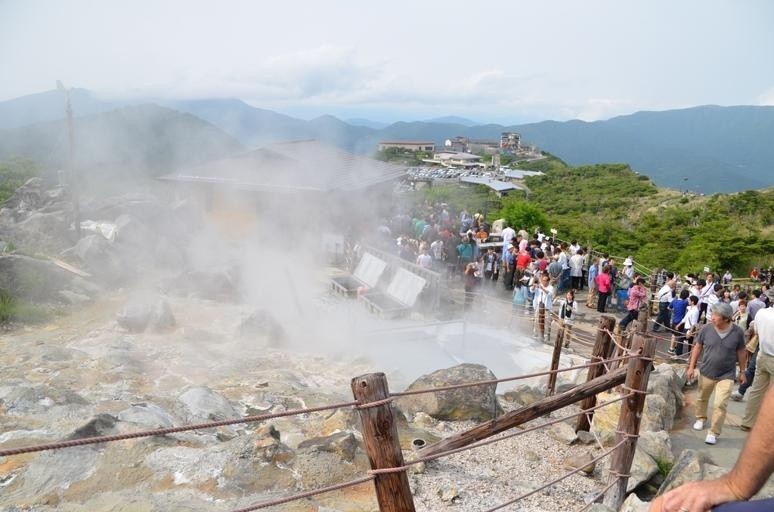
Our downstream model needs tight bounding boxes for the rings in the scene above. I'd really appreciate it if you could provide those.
[679,506,688,512]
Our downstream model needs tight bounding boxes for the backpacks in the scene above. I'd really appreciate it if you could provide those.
[472,214,481,226]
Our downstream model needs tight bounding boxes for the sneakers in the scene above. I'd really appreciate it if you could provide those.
[705,434,717,445]
[693,420,703,430]
[668,346,675,353]
[731,390,743,401]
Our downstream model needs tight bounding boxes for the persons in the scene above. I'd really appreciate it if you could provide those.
[652,264,774,446]
[645,375,774,512]
[378,201,650,332]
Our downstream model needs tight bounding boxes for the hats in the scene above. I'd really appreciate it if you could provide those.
[623,259,632,265]
[712,303,733,318]
[507,245,513,249]
[760,294,768,302]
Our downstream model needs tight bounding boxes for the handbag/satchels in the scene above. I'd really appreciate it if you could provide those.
[746,335,759,353]
